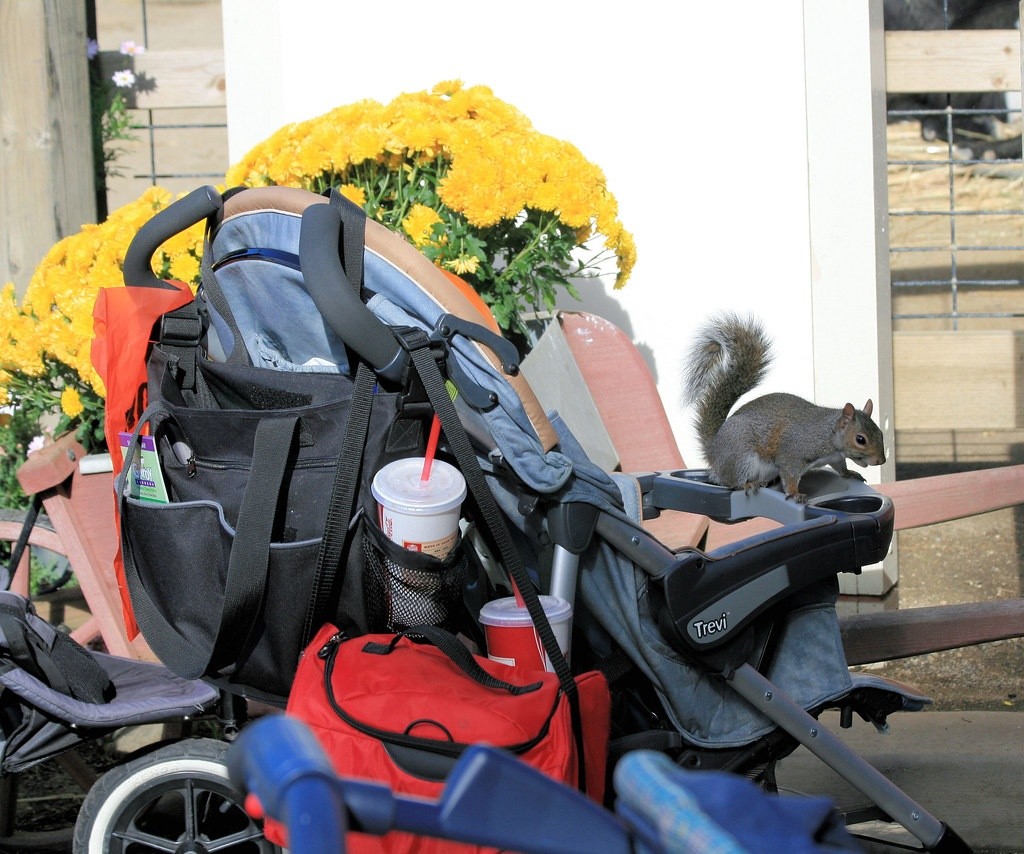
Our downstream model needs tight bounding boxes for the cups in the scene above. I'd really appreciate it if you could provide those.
[370,457,466,627]
[480,595,573,672]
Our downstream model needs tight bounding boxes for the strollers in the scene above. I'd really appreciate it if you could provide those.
[0,182,981,854]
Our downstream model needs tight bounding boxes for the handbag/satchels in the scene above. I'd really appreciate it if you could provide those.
[116,295,497,713]
[244,325,610,854]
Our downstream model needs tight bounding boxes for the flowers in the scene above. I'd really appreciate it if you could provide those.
[0,80,637,454]
[85,37,145,189]
